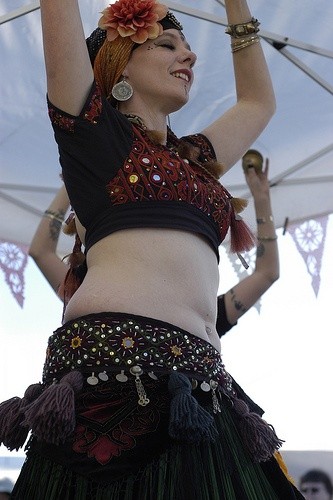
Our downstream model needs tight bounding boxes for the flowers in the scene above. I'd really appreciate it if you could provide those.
[98,0,169,44]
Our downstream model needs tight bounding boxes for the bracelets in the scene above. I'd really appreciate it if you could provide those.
[231,35,260,53]
[257,235,277,240]
[45,210,64,223]
[225,16,261,37]
[257,216,273,223]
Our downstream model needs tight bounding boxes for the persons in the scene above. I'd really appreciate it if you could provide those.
[0,1,305,500]
[28,157,280,339]
[300,469,333,500]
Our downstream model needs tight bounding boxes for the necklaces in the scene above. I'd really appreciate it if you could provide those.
[124,112,179,157]
